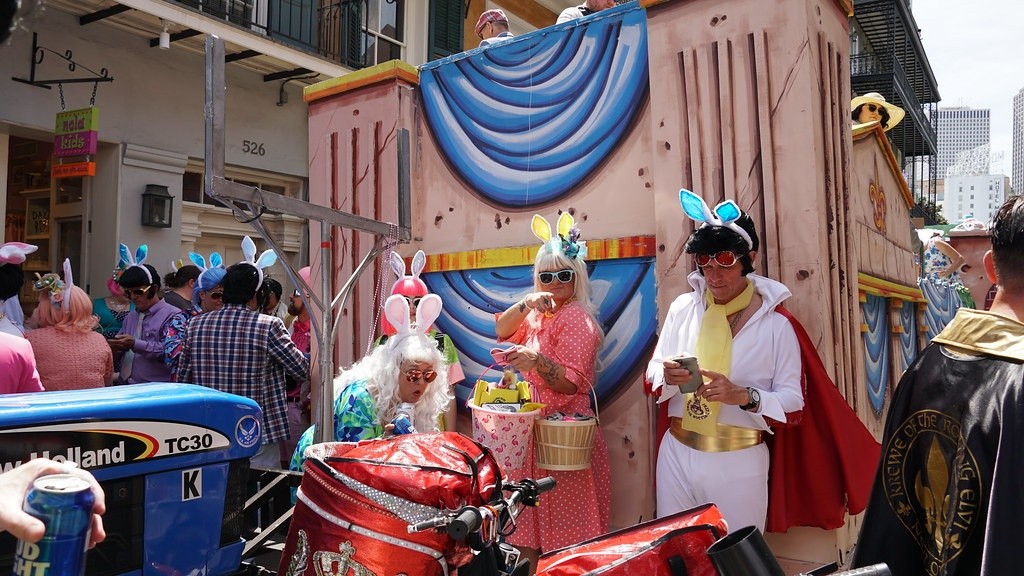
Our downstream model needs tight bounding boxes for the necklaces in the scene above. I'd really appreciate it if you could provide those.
[728,311,742,329]
[545,298,573,318]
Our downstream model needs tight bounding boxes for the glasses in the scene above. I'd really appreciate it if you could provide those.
[398,368,438,384]
[204,290,223,300]
[123,285,152,296]
[863,103,887,116]
[538,270,575,285]
[293,289,309,298]
[477,21,492,38]
[692,250,744,267]
[404,296,421,308]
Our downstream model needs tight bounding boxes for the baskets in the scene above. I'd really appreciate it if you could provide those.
[278,442,505,576]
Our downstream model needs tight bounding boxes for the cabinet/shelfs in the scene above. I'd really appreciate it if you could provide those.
[18,188,51,318]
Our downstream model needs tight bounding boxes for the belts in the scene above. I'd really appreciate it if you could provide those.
[286,396,302,403]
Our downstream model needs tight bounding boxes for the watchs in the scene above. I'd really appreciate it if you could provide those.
[739,387,760,410]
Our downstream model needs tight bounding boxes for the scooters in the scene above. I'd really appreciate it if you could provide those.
[274,432,788,576]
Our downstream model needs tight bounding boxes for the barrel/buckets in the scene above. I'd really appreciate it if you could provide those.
[464,359,541,472]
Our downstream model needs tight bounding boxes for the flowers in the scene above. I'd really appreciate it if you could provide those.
[50,279,66,309]
[556,221,581,258]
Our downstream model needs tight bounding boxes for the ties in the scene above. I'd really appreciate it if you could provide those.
[120,313,146,381]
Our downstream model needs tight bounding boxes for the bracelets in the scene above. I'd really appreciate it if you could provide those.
[524,293,535,311]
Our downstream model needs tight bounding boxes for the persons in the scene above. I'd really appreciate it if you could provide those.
[851,191,1024,576]
[645,209,804,536]
[176,262,310,539]
[274,284,310,536]
[475,8,516,48]
[93,270,136,385]
[556,0,615,25]
[107,263,187,384]
[851,92,906,133]
[23,280,114,392]
[161,266,227,382]
[288,328,455,514]
[922,237,965,286]
[495,235,613,576]
[0,331,45,394]
[256,280,297,338]
[373,277,466,431]
[159,272,179,298]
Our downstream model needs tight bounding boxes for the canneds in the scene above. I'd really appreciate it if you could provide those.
[390,415,418,434]
[12,474,95,576]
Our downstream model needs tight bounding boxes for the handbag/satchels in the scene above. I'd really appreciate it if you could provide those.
[324,428,505,507]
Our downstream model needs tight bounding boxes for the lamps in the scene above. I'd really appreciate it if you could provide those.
[159,20,176,49]
[140,184,175,227]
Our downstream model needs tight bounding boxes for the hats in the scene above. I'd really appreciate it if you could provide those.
[474,9,508,35]
[851,92,905,133]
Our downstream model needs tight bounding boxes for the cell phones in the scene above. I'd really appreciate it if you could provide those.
[673,356,704,394]
